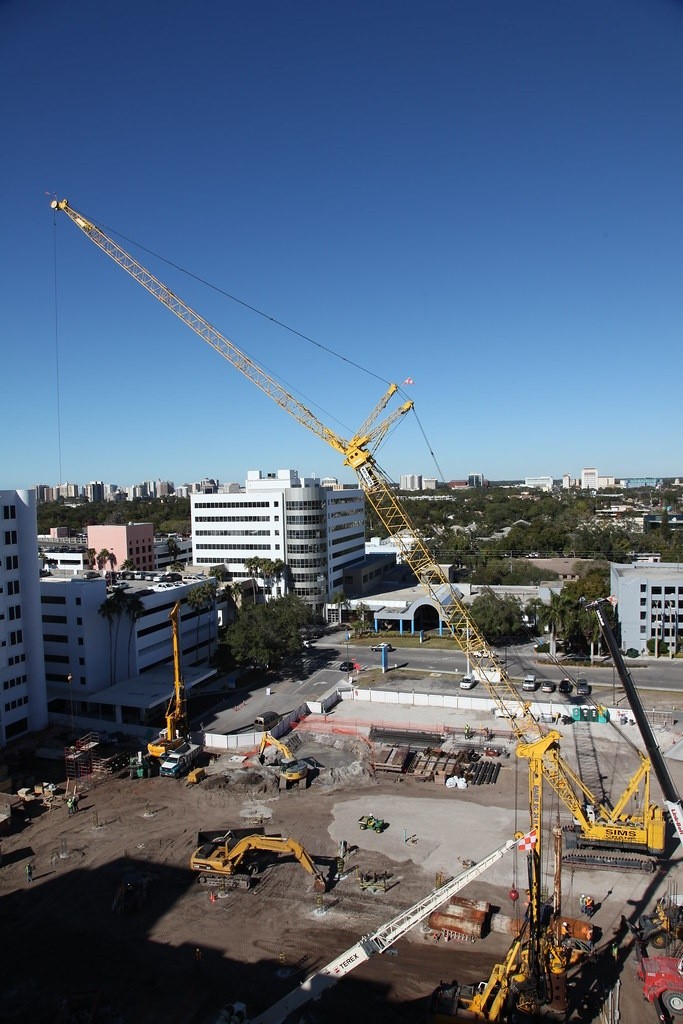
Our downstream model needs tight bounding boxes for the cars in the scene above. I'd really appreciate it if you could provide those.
[559,680,570,693]
[541,681,553,692]
[339,662,354,671]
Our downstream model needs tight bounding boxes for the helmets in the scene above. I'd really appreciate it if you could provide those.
[582,895,584,898]
[69,799,71,802]
[563,922,567,926]
[587,896,590,900]
[73,796,76,799]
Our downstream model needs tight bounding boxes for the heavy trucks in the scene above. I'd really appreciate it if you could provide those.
[159,745,203,779]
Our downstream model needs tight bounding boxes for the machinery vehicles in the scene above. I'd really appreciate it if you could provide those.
[466,730,602,1024]
[358,816,384,834]
[577,595,683,1014]
[50,201,665,874]
[148,603,189,758]
[190,830,328,890]
[257,731,308,790]
[213,828,536,1024]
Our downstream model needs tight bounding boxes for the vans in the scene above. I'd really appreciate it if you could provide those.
[254,711,283,732]
[522,675,535,691]
[459,674,475,689]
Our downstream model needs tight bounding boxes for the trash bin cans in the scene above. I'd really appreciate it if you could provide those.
[572,707,608,723]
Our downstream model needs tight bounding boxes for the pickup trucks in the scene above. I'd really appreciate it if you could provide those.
[473,650,495,658]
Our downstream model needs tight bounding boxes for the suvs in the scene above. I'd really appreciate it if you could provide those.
[577,679,588,694]
[371,642,392,652]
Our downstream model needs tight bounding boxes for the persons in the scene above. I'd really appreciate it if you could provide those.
[561,922,569,935]
[374,873,379,882]
[67,799,75,814]
[25,863,33,883]
[193,947,203,976]
[359,872,364,883]
[73,799,79,813]
